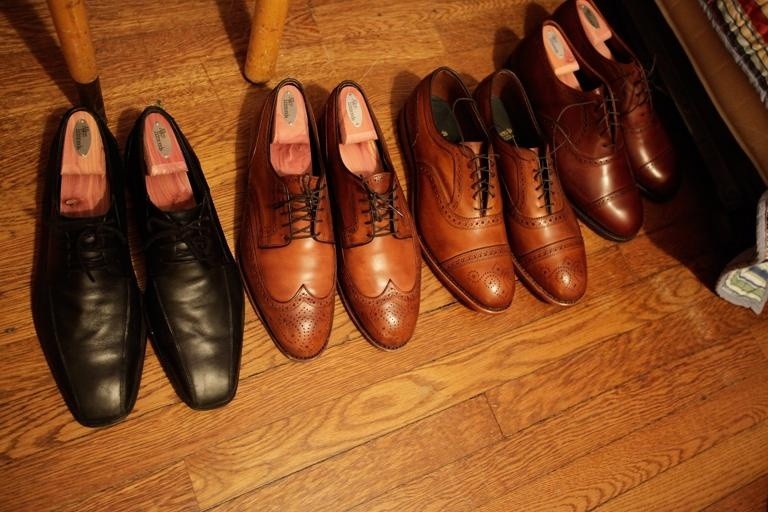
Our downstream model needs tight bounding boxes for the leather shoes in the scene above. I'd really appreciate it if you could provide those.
[472,70,588,308]
[319,79,421,352]
[41,106,148,427]
[237,78,338,361]
[398,66,515,316]
[125,106,244,411]
